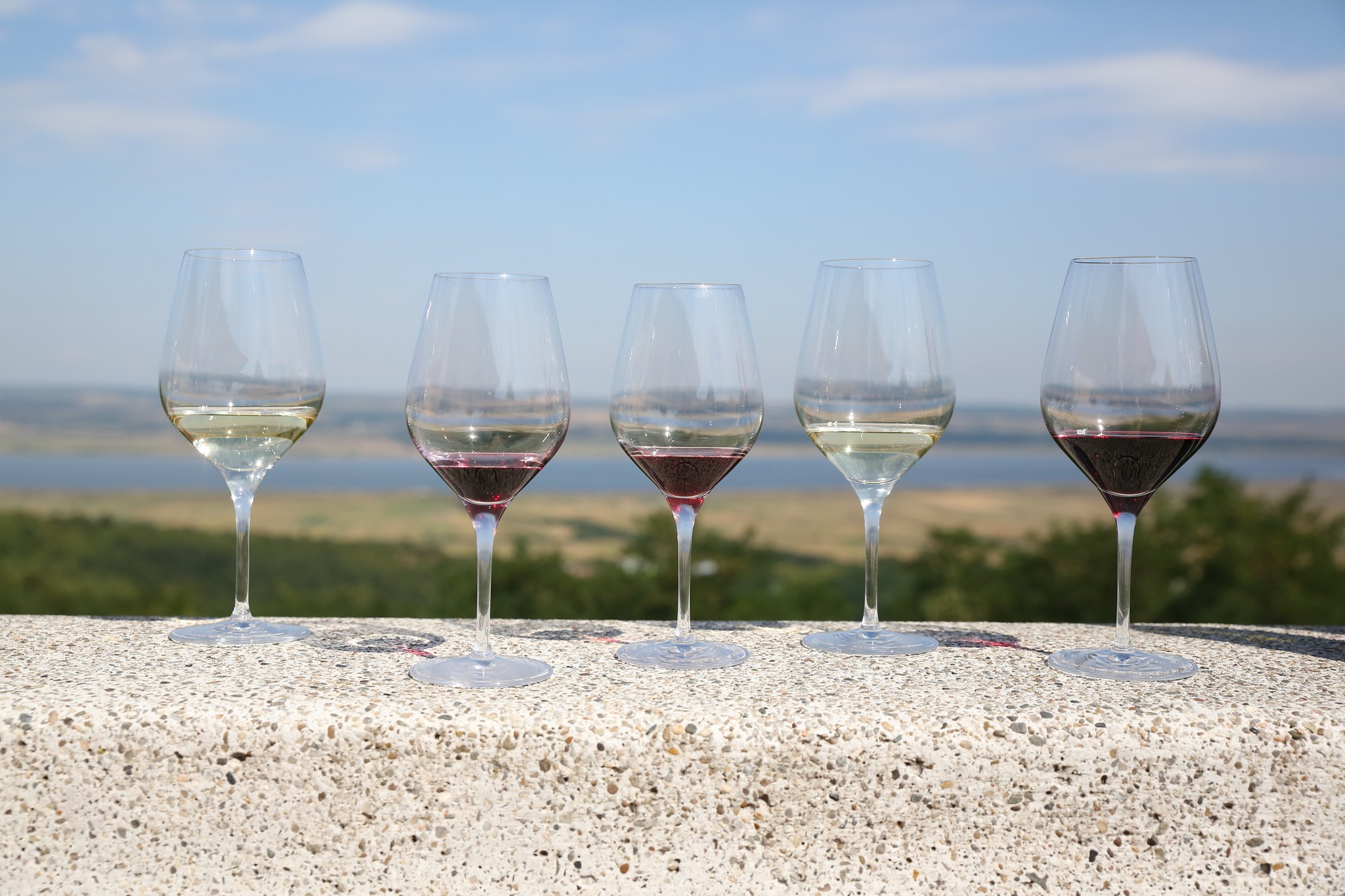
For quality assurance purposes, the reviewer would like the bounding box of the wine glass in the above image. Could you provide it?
[404,271,572,689]
[157,248,327,648]
[1040,255,1223,682]
[793,258,956,656]
[610,282,765,671]
